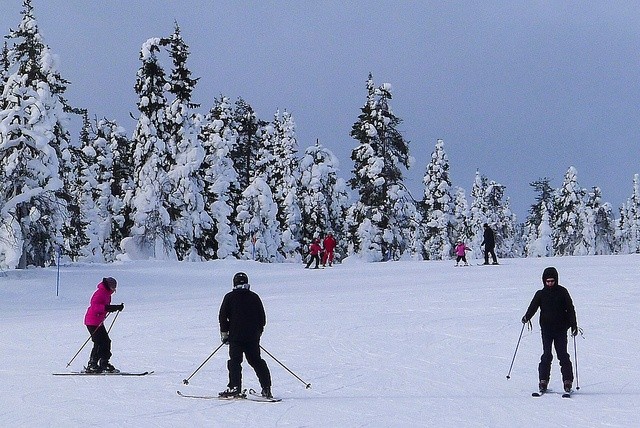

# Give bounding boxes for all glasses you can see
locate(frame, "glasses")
[546,280,554,282]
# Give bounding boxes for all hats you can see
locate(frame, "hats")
[328,230,332,234]
[456,240,462,243]
[234,272,248,287]
[107,277,117,288]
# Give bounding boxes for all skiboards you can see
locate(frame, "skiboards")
[477,264,509,266]
[310,268,324,269]
[177,391,282,402]
[52,371,154,376]
[454,265,473,267]
[532,389,574,398]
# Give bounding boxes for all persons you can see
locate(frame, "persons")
[522,266,579,392]
[219,272,273,398]
[455,240,472,265]
[481,223,498,265]
[83,276,123,374]
[321,232,336,267]
[305,238,324,268]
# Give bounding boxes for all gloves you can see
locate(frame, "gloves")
[221,332,230,344]
[106,305,118,311]
[117,304,124,311]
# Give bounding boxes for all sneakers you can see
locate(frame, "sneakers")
[219,386,239,397]
[101,360,120,373]
[539,380,547,392]
[86,362,110,373]
[261,387,271,398]
[564,379,572,392]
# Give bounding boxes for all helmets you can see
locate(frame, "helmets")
[315,238,320,243]
